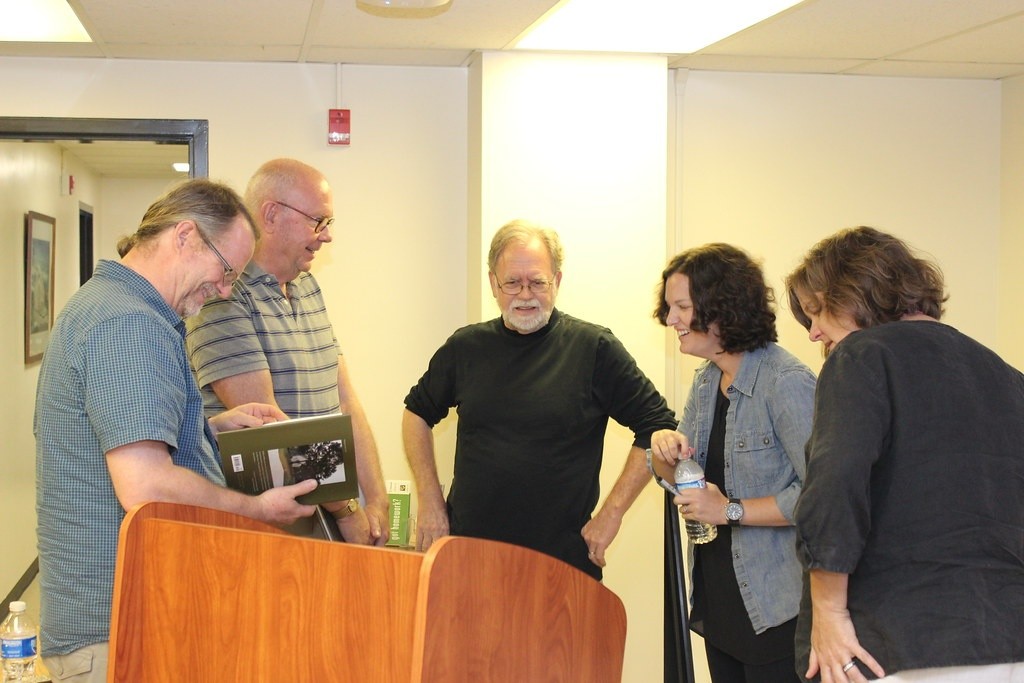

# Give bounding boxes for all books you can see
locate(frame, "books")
[216,413,359,505]
[384,480,411,548]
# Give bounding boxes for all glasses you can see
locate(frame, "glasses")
[175,220,237,287]
[276,201,335,233]
[495,272,555,295]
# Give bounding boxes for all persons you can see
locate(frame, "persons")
[32,182,319,683]
[644,244,818,683]
[185,159,392,549]
[782,226,1024,683]
[401,221,680,582]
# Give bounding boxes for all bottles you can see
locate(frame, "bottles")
[0,601,38,683]
[674,451,717,544]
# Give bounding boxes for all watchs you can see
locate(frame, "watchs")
[330,499,359,521]
[724,498,745,528]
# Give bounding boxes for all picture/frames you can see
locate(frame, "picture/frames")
[24,211,55,365]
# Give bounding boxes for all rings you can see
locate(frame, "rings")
[588,552,596,555]
[842,661,855,673]
